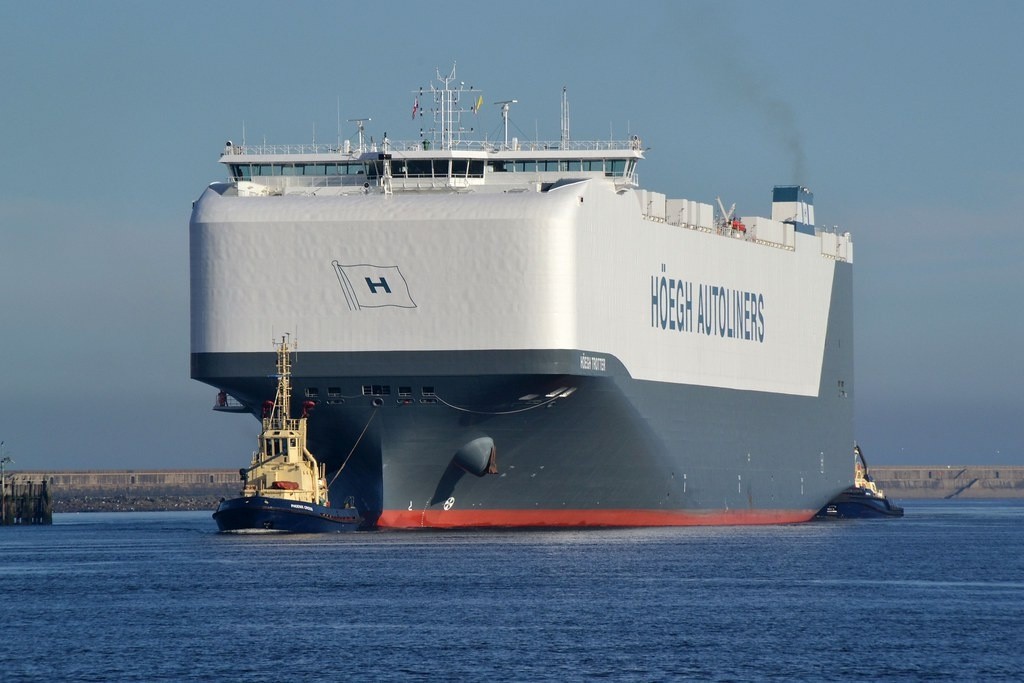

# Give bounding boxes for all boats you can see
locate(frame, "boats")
[817,438,903,521]
[213,320,362,531]
[0,440,55,526]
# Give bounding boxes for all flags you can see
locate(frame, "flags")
[412,97,418,119]
[477,95,483,110]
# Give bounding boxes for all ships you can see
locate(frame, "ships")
[188,59,856,531]
